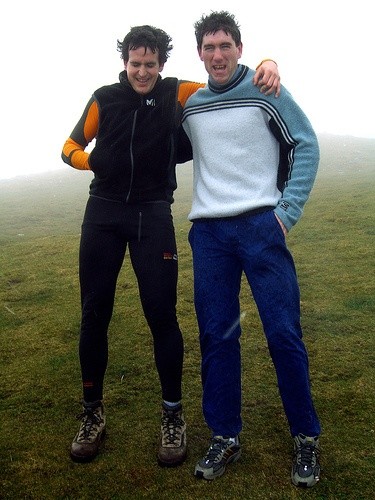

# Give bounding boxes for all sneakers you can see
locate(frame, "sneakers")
[157,401,188,467]
[194,433,243,482]
[289,430,322,490]
[68,401,107,464]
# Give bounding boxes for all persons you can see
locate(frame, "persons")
[175,12,324,489]
[60,26,283,469]
